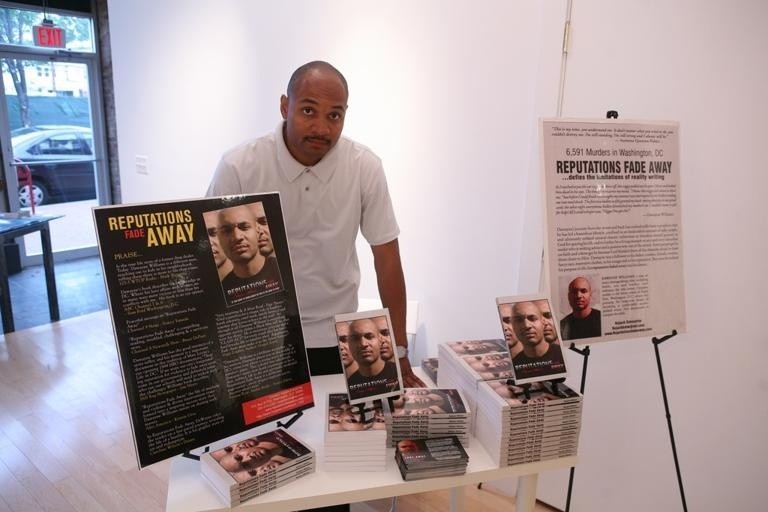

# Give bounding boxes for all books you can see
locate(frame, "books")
[199,426,315,508]
[321,386,472,481]
[422,338,584,469]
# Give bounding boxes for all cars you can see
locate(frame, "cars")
[0,125,96,208]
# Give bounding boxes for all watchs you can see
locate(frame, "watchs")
[395,346,408,360]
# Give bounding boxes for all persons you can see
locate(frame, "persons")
[369,315,396,364]
[334,321,359,380]
[204,61,429,510]
[497,303,524,360]
[561,276,601,340]
[511,301,566,380]
[347,318,400,400]
[529,298,560,346]
[244,201,276,259]
[216,204,286,306]
[202,211,233,283]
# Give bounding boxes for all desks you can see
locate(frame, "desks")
[0,212,67,334]
[166,366,577,512]
